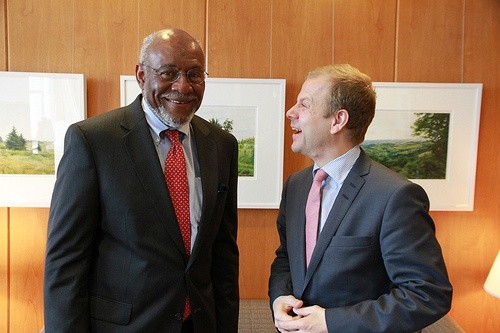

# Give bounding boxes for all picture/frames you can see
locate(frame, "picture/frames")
[0,71,87,209]
[360,82,483,211]
[119,75,286,209]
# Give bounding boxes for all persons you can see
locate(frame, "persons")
[268,63,454,333]
[43,28,239,333]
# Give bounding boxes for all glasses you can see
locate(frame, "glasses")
[143,64,209,85]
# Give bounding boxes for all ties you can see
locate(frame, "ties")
[305,169,329,273]
[161,129,191,321]
